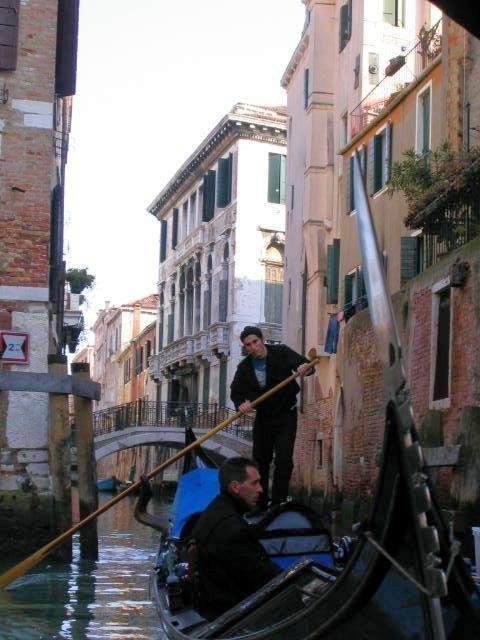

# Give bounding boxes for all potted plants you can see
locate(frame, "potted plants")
[65,268,94,293]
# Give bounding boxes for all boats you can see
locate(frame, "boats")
[132,146,477,640]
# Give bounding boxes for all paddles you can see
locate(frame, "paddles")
[0,356,321,592]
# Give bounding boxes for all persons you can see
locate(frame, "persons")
[230,326,316,518]
[190,457,272,622]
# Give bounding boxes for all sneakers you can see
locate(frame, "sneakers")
[244,501,268,519]
[269,501,281,511]
